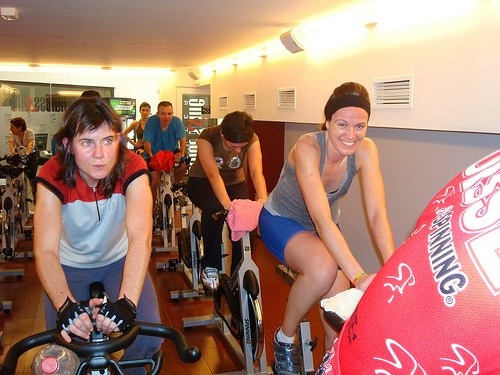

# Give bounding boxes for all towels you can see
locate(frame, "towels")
[149,150,175,173]
[319,287,364,321]
[225,198,264,242]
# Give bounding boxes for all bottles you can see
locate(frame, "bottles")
[31,344,81,375]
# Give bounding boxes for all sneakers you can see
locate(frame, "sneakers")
[202,266,219,291]
[273,326,300,375]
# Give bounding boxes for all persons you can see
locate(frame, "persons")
[142,101,186,224]
[187,111,267,291]
[32,98,164,375]
[121,102,151,157]
[8,117,40,205]
[258,82,395,375]
[51,90,127,156]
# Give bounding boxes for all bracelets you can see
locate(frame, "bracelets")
[353,272,367,285]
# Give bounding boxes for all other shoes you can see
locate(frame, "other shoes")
[175,187,183,197]
